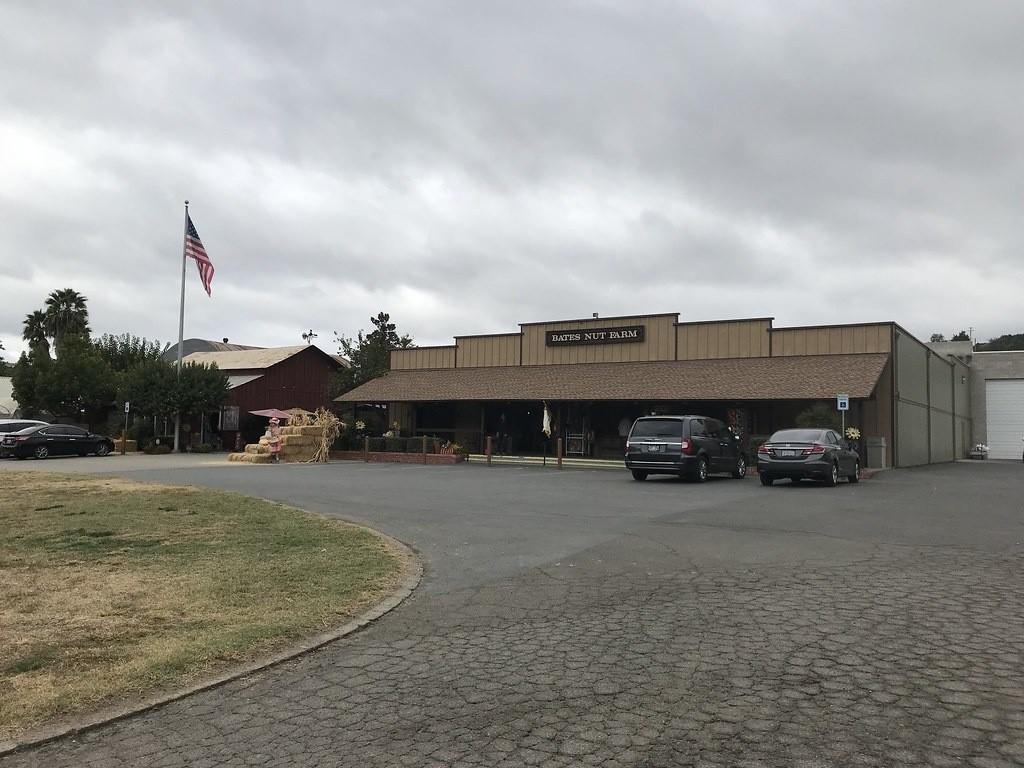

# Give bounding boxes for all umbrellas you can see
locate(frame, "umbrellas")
[250,408,292,420]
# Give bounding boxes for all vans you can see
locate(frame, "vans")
[0,419,51,445]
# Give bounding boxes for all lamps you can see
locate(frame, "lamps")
[592,313,599,318]
[962,376,966,384]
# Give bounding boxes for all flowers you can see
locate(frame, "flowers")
[846,427,860,449]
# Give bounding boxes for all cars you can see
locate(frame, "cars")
[0,423,116,460]
[756,428,861,486]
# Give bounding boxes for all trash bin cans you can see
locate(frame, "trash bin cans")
[865,436,887,468]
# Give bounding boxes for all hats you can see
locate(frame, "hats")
[269,418,280,424]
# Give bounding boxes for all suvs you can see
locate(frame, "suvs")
[625,413,747,483]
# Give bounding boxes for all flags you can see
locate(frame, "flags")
[184,216,215,297]
[541,406,552,440]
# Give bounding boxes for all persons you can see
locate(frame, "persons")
[268,417,281,460]
[618,415,631,458]
